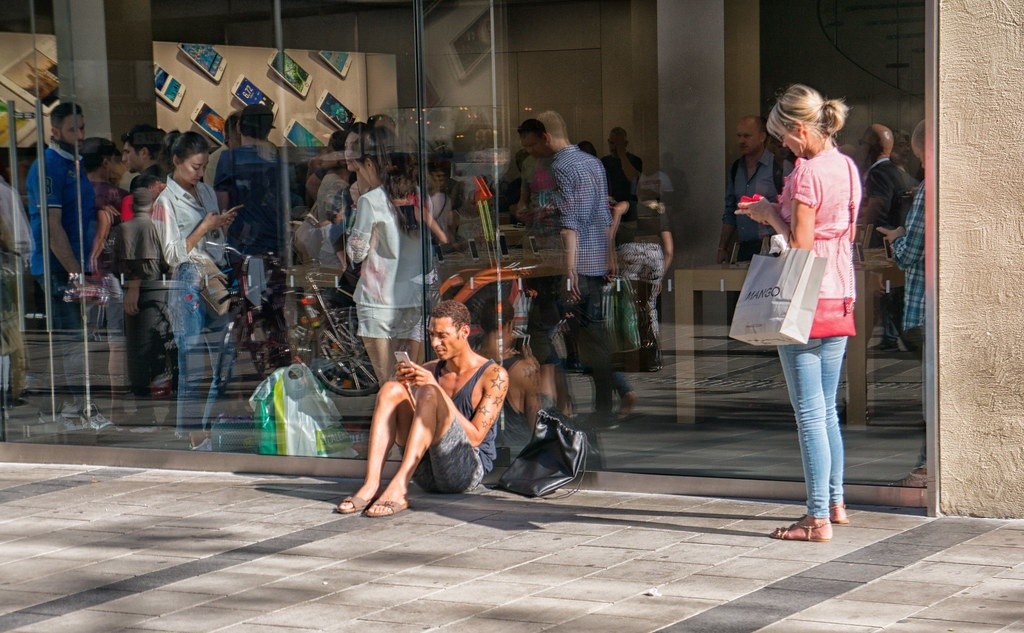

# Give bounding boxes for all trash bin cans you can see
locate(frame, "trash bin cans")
[125,281,192,400]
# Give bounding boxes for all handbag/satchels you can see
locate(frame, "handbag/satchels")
[494,407,588,500]
[194,256,232,318]
[728,235,828,345]
[247,362,360,458]
[63,268,110,305]
[601,274,641,353]
[209,414,259,453]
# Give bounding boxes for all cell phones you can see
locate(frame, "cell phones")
[855,224,893,263]
[317,51,353,79]
[267,49,313,98]
[153,62,186,110]
[435,232,568,264]
[177,43,228,83]
[394,352,412,368]
[316,89,361,131]
[231,74,278,119]
[446,7,492,81]
[283,118,327,146]
[226,204,244,214]
[730,242,740,265]
[190,100,226,146]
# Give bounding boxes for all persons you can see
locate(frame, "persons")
[203,103,282,276]
[333,299,509,518]
[81,123,165,432]
[303,113,496,387]
[0,176,35,408]
[473,298,542,473]
[528,109,638,434]
[503,118,555,223]
[110,188,170,425]
[734,84,862,542]
[609,173,672,371]
[860,123,924,367]
[575,126,643,246]
[876,123,926,488]
[26,103,96,422]
[151,128,240,451]
[717,114,793,377]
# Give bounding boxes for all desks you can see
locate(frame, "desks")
[438,211,572,303]
[673,247,905,427]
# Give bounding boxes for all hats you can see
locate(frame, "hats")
[242,102,276,129]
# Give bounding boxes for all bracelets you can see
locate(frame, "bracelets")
[719,247,727,251]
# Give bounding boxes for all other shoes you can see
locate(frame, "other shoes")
[58,411,79,427]
[868,337,899,351]
[189,436,213,453]
[79,406,115,430]
[614,391,638,422]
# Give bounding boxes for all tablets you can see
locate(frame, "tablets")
[0,37,61,115]
[0,97,37,147]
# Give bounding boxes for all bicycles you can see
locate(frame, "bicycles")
[203,239,379,396]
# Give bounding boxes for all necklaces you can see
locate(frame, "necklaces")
[357,180,371,196]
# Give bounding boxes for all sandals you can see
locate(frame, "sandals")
[889,466,928,487]
[801,504,850,523]
[768,515,833,543]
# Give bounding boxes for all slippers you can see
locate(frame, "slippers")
[335,492,380,513]
[366,497,411,517]
